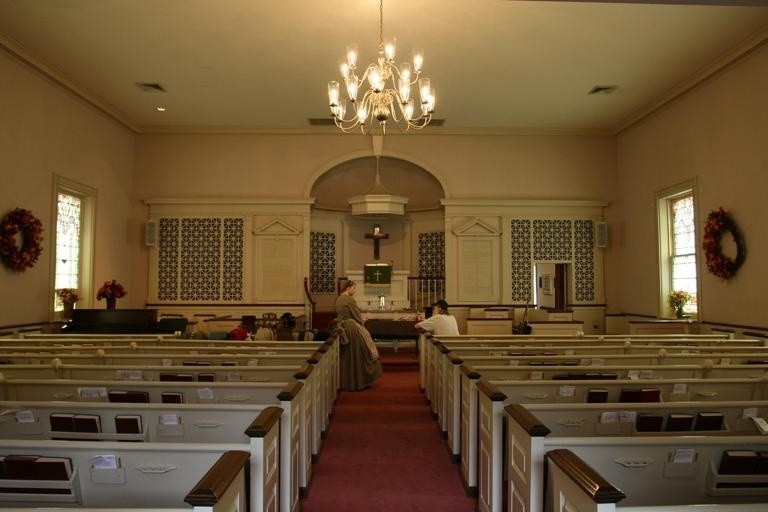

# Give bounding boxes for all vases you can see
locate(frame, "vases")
[63,303,74,320]
[675,304,685,319]
[106,298,116,310]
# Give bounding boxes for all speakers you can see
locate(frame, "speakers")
[144,220,156,247]
[596,221,608,248]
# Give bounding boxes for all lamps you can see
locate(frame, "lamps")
[346,156,409,216]
[328,0,435,136]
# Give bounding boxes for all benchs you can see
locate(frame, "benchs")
[417,328,768,512]
[0,331,340,512]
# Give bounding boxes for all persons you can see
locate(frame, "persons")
[414,299,460,335]
[334,280,384,391]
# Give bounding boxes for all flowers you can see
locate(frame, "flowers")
[0,206,45,273]
[96,278,127,301]
[55,288,83,305]
[667,291,694,312]
[702,206,746,282]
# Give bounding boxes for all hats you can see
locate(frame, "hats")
[430,299,448,309]
